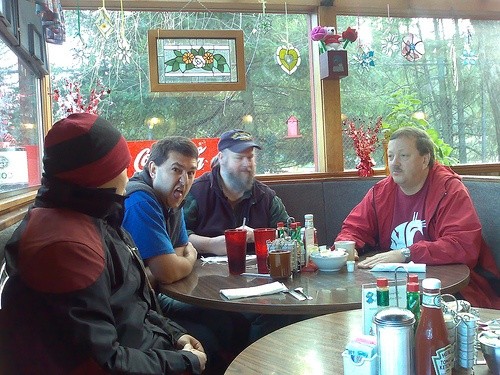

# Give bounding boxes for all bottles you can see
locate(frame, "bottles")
[371,307,416,375]
[376,278,389,311]
[406,274,421,331]
[303,214,318,271]
[276,221,306,273]
[414,278,453,375]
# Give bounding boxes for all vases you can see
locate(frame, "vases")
[319,49,348,80]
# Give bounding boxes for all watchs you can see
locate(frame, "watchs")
[401,248,410,263]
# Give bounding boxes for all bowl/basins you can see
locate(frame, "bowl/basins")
[310,251,349,271]
[477,328,500,375]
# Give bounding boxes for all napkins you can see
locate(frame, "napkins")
[200,253,256,260]
[371,263,427,272]
[219,281,284,300]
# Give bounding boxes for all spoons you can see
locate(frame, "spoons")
[294,287,313,300]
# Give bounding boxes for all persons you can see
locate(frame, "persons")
[122,136,251,375]
[181,129,324,345]
[331,127,500,310]
[1,113,208,375]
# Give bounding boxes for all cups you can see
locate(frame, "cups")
[224,229,247,275]
[342,350,377,375]
[253,228,276,274]
[268,249,292,280]
[334,241,356,265]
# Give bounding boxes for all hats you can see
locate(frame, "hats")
[42,112,131,189]
[218,130,261,153]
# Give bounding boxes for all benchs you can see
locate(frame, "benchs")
[1,175,500,273]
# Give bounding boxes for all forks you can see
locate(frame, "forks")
[280,283,306,301]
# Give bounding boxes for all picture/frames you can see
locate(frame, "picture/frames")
[148,30,246,92]
[28,24,45,65]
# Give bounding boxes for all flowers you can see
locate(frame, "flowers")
[311,25,359,53]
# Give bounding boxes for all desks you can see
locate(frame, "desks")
[225,307,500,375]
[157,251,469,314]
